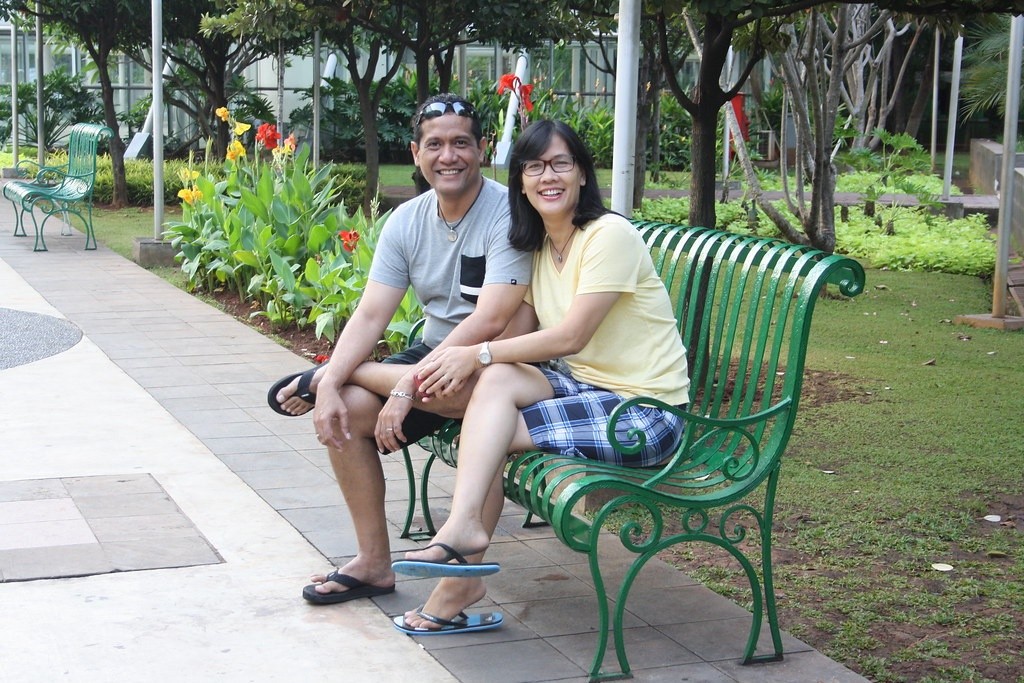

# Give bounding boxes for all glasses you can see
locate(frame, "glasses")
[416,101,474,124]
[523,154,575,177]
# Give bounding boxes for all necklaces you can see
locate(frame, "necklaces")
[439,172,484,242]
[550,227,576,262]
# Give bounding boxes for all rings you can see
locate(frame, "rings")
[316,433,320,437]
[385,428,393,431]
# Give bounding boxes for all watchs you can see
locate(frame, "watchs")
[479,341,492,367]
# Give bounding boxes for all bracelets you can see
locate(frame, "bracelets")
[389,391,416,401]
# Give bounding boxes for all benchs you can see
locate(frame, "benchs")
[403,216,865,683]
[3,121,116,253]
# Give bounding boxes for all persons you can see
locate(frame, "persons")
[267,97,533,603]
[393,117,691,636]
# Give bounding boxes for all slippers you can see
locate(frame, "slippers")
[268,359,329,416]
[302,570,395,604]
[391,543,500,578]
[393,604,504,635]
